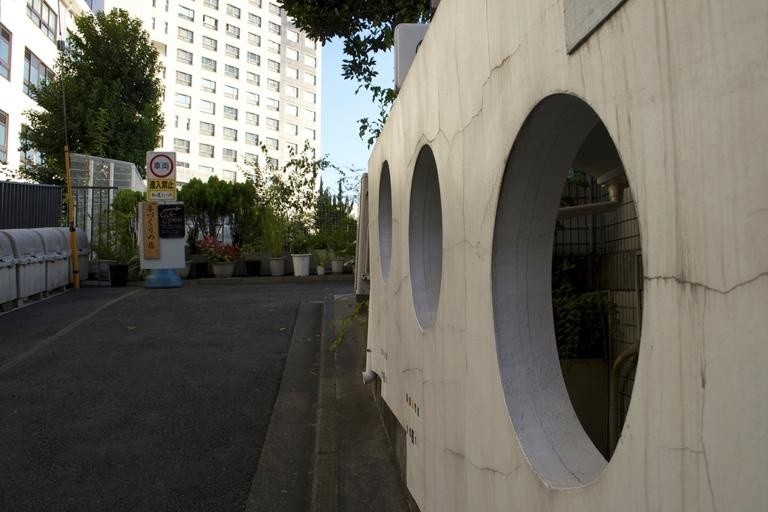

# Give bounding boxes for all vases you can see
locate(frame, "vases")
[212,261,234,277]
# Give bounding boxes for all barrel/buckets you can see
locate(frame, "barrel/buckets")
[245,260,261,275]
[291,254,312,276]
[332,260,344,273]
[270,257,285,276]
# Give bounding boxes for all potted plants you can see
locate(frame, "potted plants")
[90,234,116,281]
[267,223,346,277]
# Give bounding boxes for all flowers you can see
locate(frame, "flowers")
[200,236,240,264]
